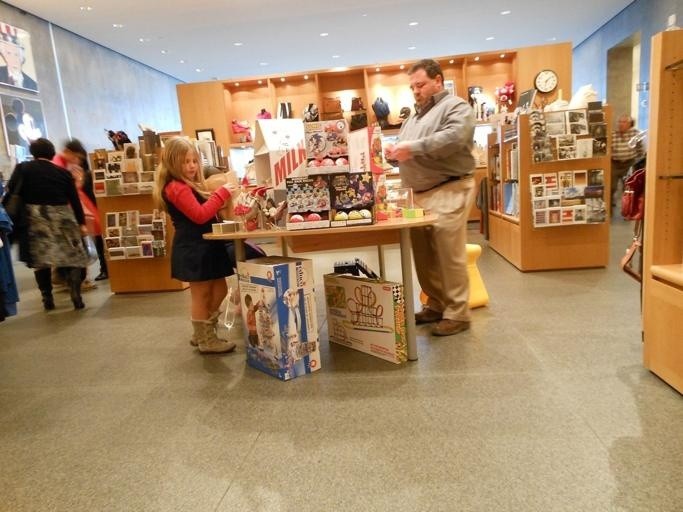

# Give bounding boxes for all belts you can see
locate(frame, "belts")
[408,171,472,195]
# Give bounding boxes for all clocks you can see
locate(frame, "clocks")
[532,68,559,95]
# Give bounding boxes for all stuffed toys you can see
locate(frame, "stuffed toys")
[496,81,515,107]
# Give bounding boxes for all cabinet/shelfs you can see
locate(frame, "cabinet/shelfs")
[85,144,188,294]
[485,110,612,273]
[175,42,571,222]
[641,27,683,401]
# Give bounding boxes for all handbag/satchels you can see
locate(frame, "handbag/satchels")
[5,162,26,216]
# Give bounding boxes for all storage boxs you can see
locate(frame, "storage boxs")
[235,254,321,381]
[323,273,409,364]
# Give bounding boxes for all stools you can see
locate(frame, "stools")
[421,245,491,311]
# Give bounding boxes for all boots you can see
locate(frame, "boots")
[191,320,197,346]
[34,268,56,312]
[68,266,86,310]
[191,319,236,354]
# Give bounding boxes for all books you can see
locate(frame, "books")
[102,235,120,249]
[568,107,589,135]
[492,147,501,180]
[104,212,119,227]
[535,208,547,227]
[124,246,143,258]
[105,226,121,236]
[503,124,518,141]
[151,228,164,241]
[543,173,557,186]
[137,213,155,225]
[104,178,121,196]
[135,235,155,246]
[576,137,593,158]
[141,170,154,193]
[588,168,605,183]
[592,123,608,156]
[560,185,586,206]
[573,204,587,224]
[490,182,502,212]
[121,170,140,194]
[560,207,574,226]
[505,142,519,180]
[573,169,588,186]
[91,148,108,170]
[151,220,166,230]
[532,185,547,198]
[546,194,561,207]
[140,240,154,257]
[587,100,603,118]
[544,111,567,137]
[555,133,576,160]
[122,225,138,236]
[557,170,573,188]
[106,247,126,260]
[588,110,603,133]
[547,188,560,196]
[123,142,139,160]
[136,225,154,236]
[91,169,106,181]
[151,239,166,257]
[105,161,121,180]
[585,184,606,223]
[530,173,541,185]
[504,180,521,219]
[532,198,548,214]
[93,180,106,197]
[126,211,141,227]
[116,211,130,228]
[548,207,562,227]
[118,236,137,246]
[107,151,124,162]
[121,158,143,175]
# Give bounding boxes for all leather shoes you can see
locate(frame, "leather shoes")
[95,270,108,280]
[432,317,472,338]
[414,308,442,325]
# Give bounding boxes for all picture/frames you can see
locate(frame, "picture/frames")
[0,93,53,159]
[0,20,41,95]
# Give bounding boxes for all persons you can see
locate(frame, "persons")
[385,58,476,337]
[610,114,648,209]
[0,22,37,91]
[243,293,261,349]
[59,137,110,281]
[5,137,87,313]
[153,134,237,356]
